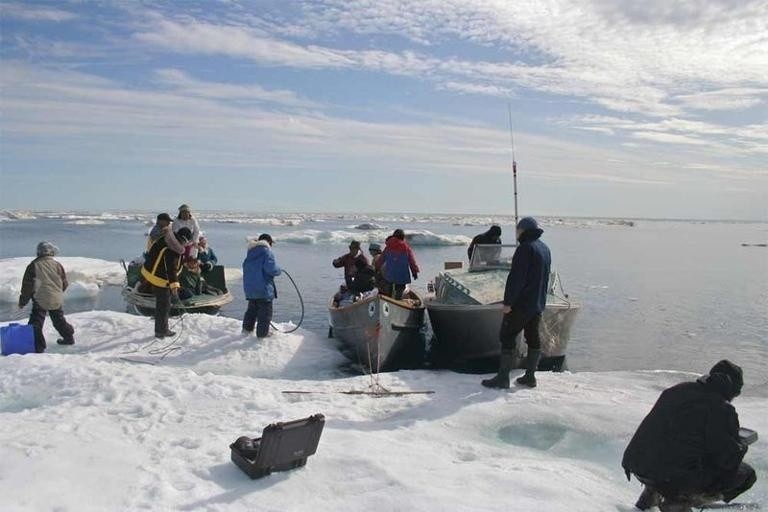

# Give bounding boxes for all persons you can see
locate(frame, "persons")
[621,358,757,512]
[331,228,420,308]
[480,218,552,389]
[241,233,282,338]
[140,226,193,339]
[468,224,503,264]
[17,240,75,354]
[138,205,218,300]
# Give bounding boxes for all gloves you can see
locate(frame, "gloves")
[200,264,211,272]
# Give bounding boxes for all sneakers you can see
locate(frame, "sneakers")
[57,336,75,345]
[242,328,276,340]
[155,330,176,338]
[658,497,694,512]
[635,484,663,510]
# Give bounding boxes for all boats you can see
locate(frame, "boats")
[423,104,574,373]
[117,256,233,317]
[327,278,428,373]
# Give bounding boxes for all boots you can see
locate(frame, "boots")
[482,349,517,389]
[517,347,543,387]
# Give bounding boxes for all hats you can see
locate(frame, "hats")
[37,242,58,255]
[157,213,174,222]
[710,360,744,392]
[199,236,207,241]
[369,243,382,251]
[178,204,190,212]
[351,240,361,248]
[177,227,193,243]
[517,216,539,230]
[258,233,276,245]
